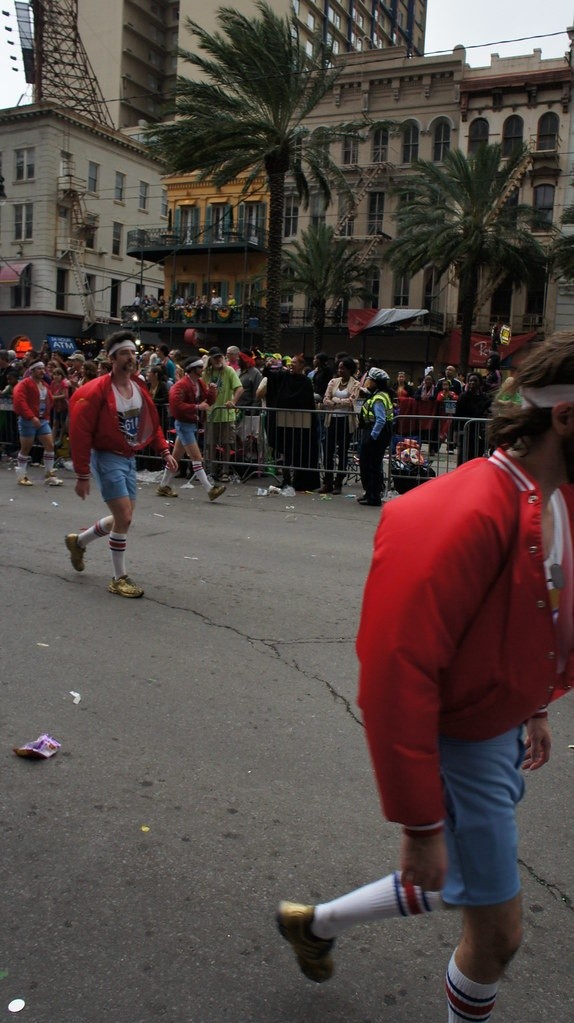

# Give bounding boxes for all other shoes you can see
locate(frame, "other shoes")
[332,488,342,494]
[359,497,382,506]
[318,485,332,493]
[221,472,229,482]
[174,472,187,478]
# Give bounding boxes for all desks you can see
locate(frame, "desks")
[160,234,180,246]
[223,230,242,242]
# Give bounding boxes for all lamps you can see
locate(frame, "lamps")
[15,251,23,259]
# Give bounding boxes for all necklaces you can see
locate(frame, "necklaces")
[340,380,348,385]
[540,502,564,589]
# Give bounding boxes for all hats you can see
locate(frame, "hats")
[68,354,85,362]
[368,366,391,381]
[209,348,223,357]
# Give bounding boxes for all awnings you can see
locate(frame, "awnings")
[0,263,31,287]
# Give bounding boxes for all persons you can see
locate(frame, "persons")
[13,358,64,486]
[135,343,500,494]
[0,340,110,461]
[158,356,227,502]
[64,330,179,598]
[355,366,394,507]
[132,292,237,324]
[277,326,574,1023]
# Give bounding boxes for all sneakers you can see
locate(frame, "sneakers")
[208,486,226,500]
[157,486,178,498]
[277,901,333,984]
[16,477,33,486]
[65,533,85,572]
[44,476,64,486]
[108,575,144,598]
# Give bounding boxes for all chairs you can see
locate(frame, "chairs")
[145,233,159,246]
[383,435,436,494]
[216,416,267,480]
[333,442,361,486]
